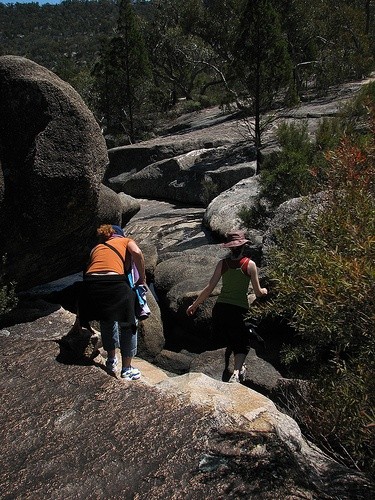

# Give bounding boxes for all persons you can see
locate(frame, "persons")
[187,232,267,385]
[83,227,146,380]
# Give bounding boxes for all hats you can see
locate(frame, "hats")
[111,225,124,235]
[223,230,249,248]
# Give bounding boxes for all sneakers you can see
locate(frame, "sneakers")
[229,363,248,383]
[106,355,118,368]
[120,367,141,380]
